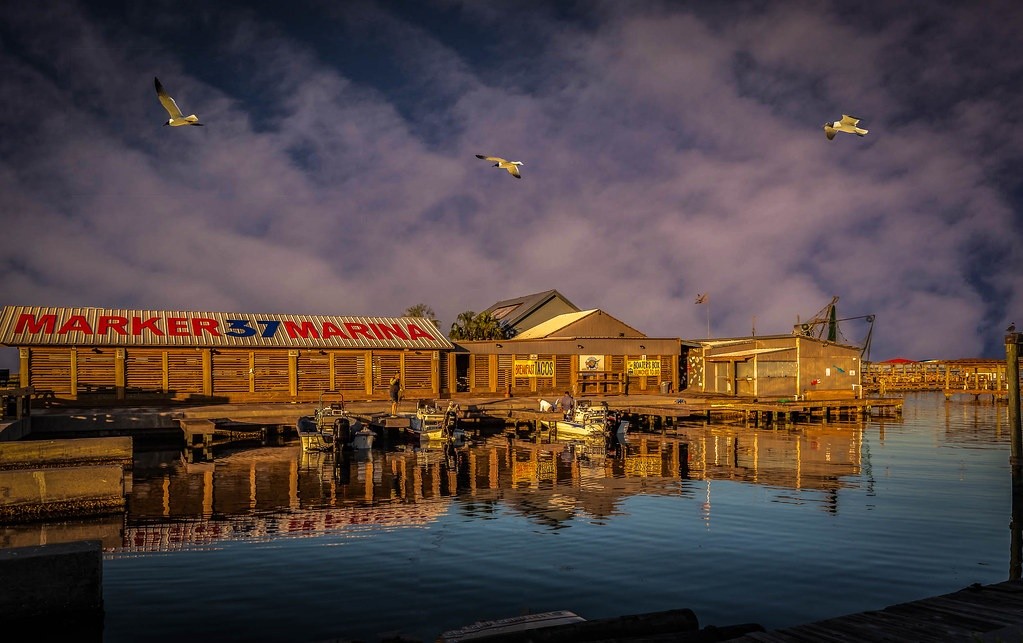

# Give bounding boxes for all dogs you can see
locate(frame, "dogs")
[537,399,554,414]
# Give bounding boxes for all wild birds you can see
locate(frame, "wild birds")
[475,154,524,179]
[154,76,205,128]
[823,115,868,140]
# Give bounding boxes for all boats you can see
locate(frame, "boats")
[295,392,378,459]
[543,397,640,448]
[411,397,498,449]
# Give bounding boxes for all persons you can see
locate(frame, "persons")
[537,399,553,413]
[390,373,401,415]
[561,391,573,420]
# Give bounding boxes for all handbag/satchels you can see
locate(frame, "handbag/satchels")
[567,410,572,415]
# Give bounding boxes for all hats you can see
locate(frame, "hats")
[395,371,400,374]
[537,399,541,402]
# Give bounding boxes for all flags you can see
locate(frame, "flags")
[696,292,709,305]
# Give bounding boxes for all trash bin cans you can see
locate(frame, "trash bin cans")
[793,395,797,401]
[660,381,668,393]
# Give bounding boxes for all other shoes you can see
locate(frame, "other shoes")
[391,414,397,416]
